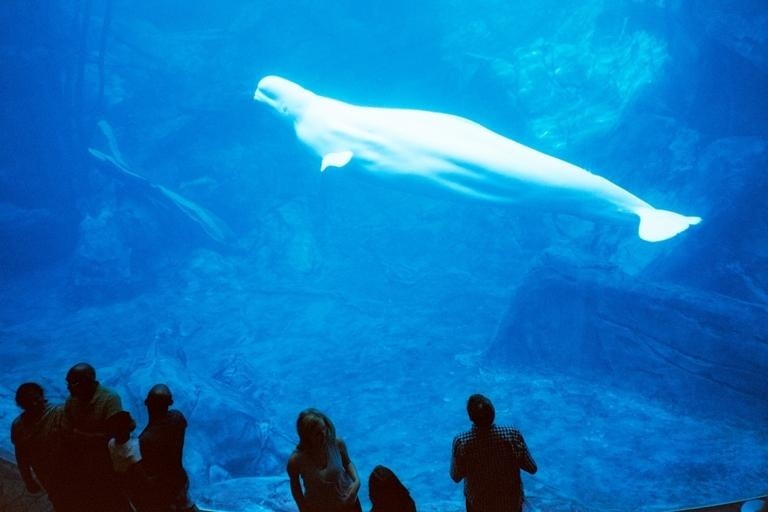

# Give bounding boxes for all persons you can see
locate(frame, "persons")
[11,363,189,512]
[287,408,363,512]
[367,464,416,512]
[449,394,538,511]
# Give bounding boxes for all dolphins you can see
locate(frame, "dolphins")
[252,74,702,244]
